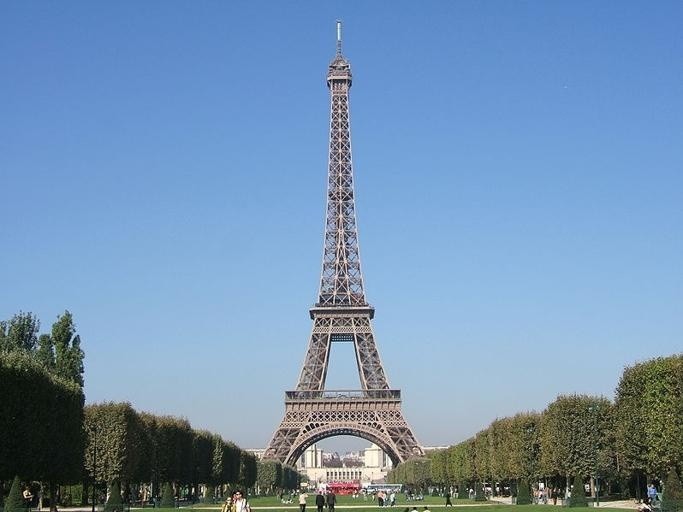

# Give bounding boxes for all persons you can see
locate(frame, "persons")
[568,489,571,498]
[585,486,590,496]
[221,491,250,512]
[107,486,151,503]
[352,488,396,507]
[299,491,308,512]
[638,478,664,512]
[316,487,337,512]
[446,490,452,508]
[469,487,473,498]
[404,485,424,502]
[23,486,34,512]
[277,488,296,504]
[404,506,431,512]
[533,487,558,505]
